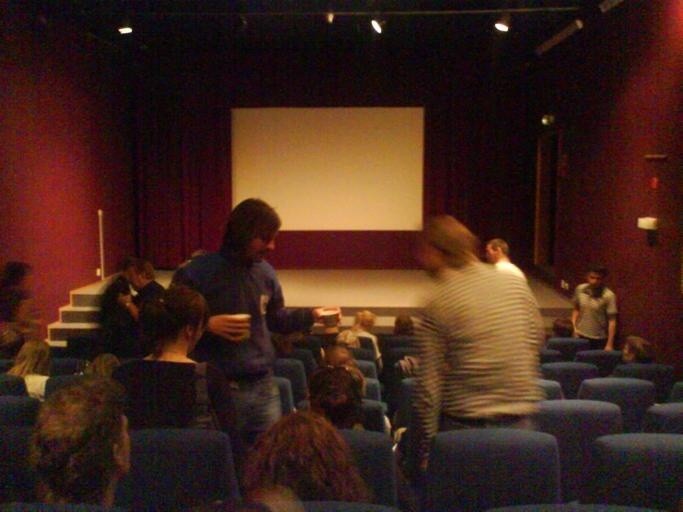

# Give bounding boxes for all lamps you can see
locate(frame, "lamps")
[111,7,579,41]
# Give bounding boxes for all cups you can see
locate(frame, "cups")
[228,314,252,341]
[320,309,338,328]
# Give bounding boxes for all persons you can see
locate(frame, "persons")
[552,318,573,337]
[394,215,544,476]
[220,307,417,511]
[622,336,656,364]
[1,199,285,511]
[570,262,618,351]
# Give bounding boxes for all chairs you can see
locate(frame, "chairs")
[0,329,681,512]
